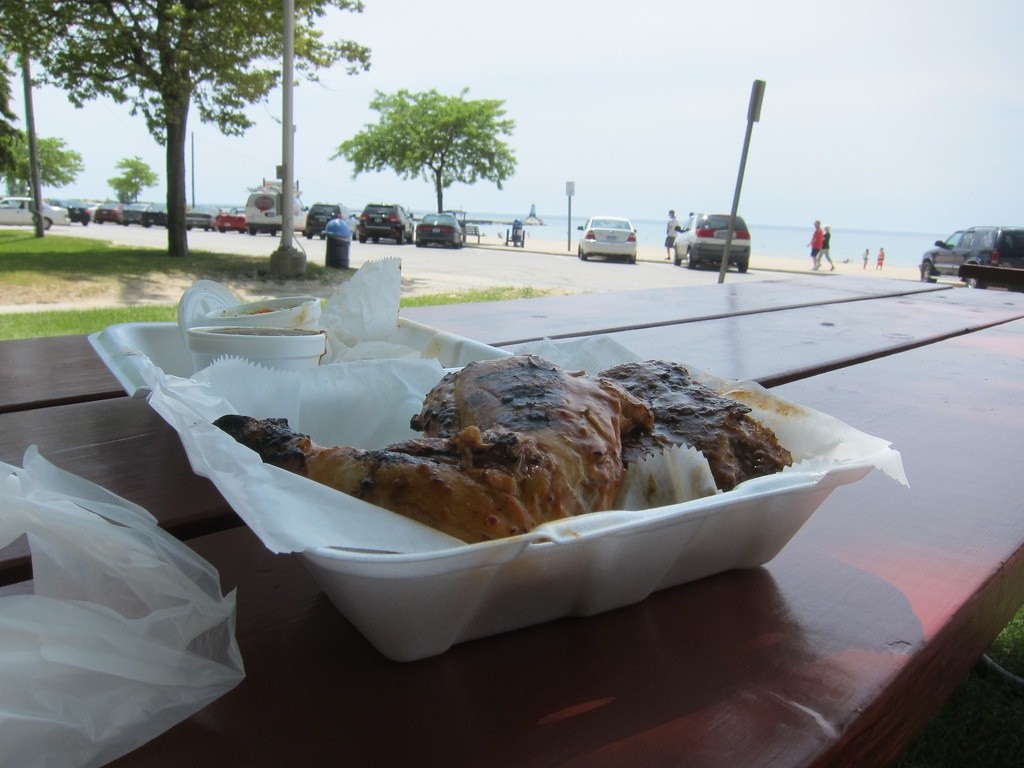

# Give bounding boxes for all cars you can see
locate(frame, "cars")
[0,197,73,231]
[186,205,224,231]
[143,203,170,229]
[87,201,104,222]
[123,203,150,228]
[576,216,638,264]
[49,199,90,226]
[96,203,126,225]
[415,214,463,250]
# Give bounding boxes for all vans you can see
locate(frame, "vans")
[244,188,311,237]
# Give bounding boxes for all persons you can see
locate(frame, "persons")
[876,248,885,270]
[817,226,836,271]
[863,249,869,270]
[807,220,825,270]
[664,210,680,259]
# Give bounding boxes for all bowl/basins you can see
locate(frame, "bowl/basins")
[198,295,325,338]
[186,322,330,381]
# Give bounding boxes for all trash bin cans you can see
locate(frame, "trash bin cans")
[320,218,352,268]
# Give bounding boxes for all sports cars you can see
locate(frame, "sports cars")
[216,207,250,233]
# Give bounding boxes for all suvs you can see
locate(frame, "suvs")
[919,225,1024,295]
[671,213,752,272]
[357,202,416,245]
[305,202,360,241]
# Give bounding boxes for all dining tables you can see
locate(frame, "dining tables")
[1,275,1024,768]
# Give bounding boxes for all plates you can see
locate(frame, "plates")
[82,315,884,665]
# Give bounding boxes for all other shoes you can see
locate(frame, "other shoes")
[830,266,835,272]
[665,258,671,260]
